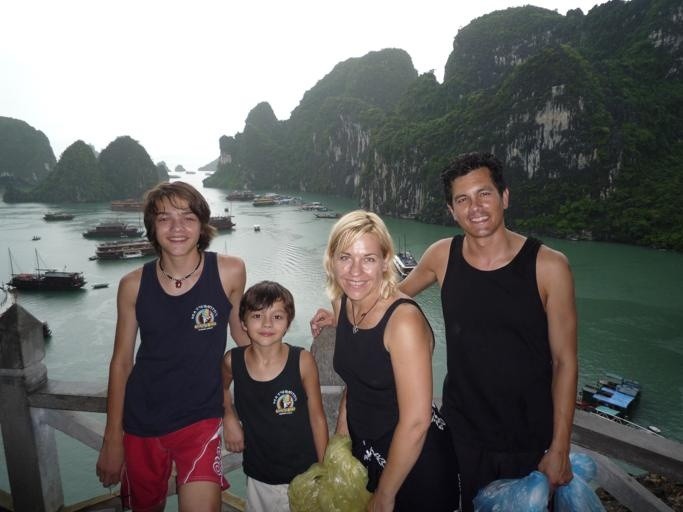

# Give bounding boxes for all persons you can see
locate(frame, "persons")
[309,151,579,512]
[97,181,250,512]
[324,210,460,512]
[222,280,329,512]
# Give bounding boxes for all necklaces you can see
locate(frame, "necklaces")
[160,251,202,288]
[352,297,380,337]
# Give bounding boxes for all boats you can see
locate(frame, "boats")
[43,199,236,260]
[393,232,418,279]
[225,189,341,219]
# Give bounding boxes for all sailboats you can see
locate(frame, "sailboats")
[5,247,88,290]
